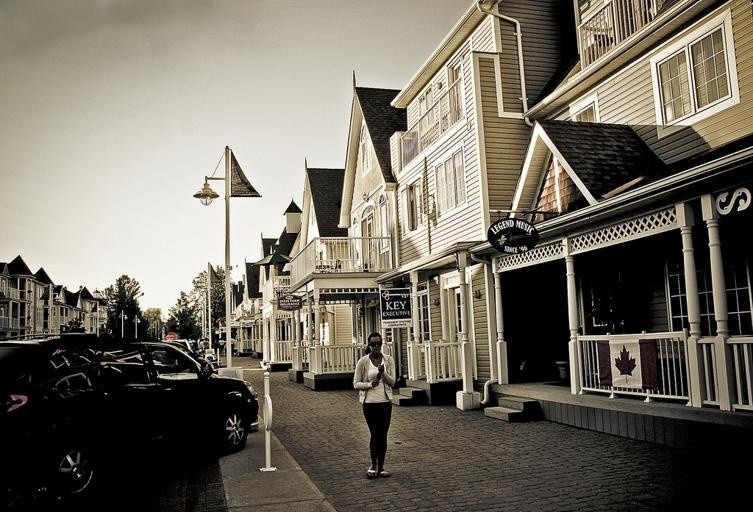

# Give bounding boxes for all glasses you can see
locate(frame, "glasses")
[369,342,382,347]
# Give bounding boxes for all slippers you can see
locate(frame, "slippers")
[368,465,392,478]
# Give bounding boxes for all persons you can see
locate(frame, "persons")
[354,332,396,478]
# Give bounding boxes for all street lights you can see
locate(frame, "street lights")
[194,146,231,368]
